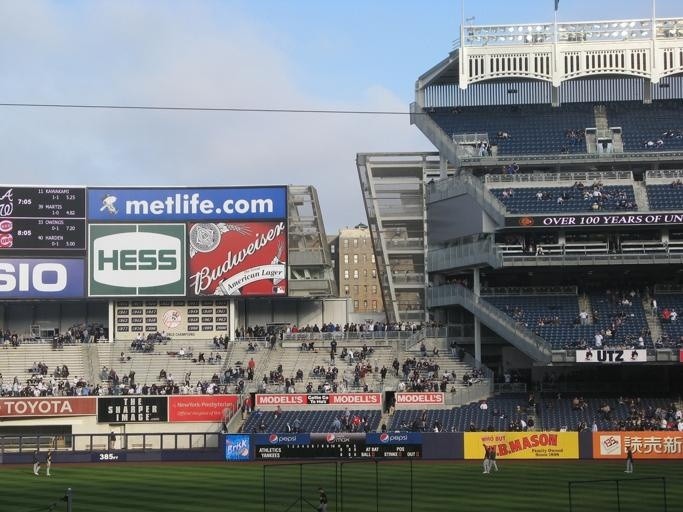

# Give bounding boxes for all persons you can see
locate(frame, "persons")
[108,429,117,451]
[501,178,638,211]
[500,162,520,174]
[481,443,489,475]
[562,128,585,146]
[638,126,682,150]
[495,128,510,140]
[622,446,634,474]
[30,450,41,476]
[476,140,485,156]
[676,179,681,185]
[670,180,676,188]
[486,143,492,156]
[316,486,327,511]
[488,448,498,473]
[43,451,53,477]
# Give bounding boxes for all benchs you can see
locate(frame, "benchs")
[0,338,416,437]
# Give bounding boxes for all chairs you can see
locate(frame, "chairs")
[418,100,682,431]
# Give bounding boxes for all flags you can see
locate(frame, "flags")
[553,1,559,13]
